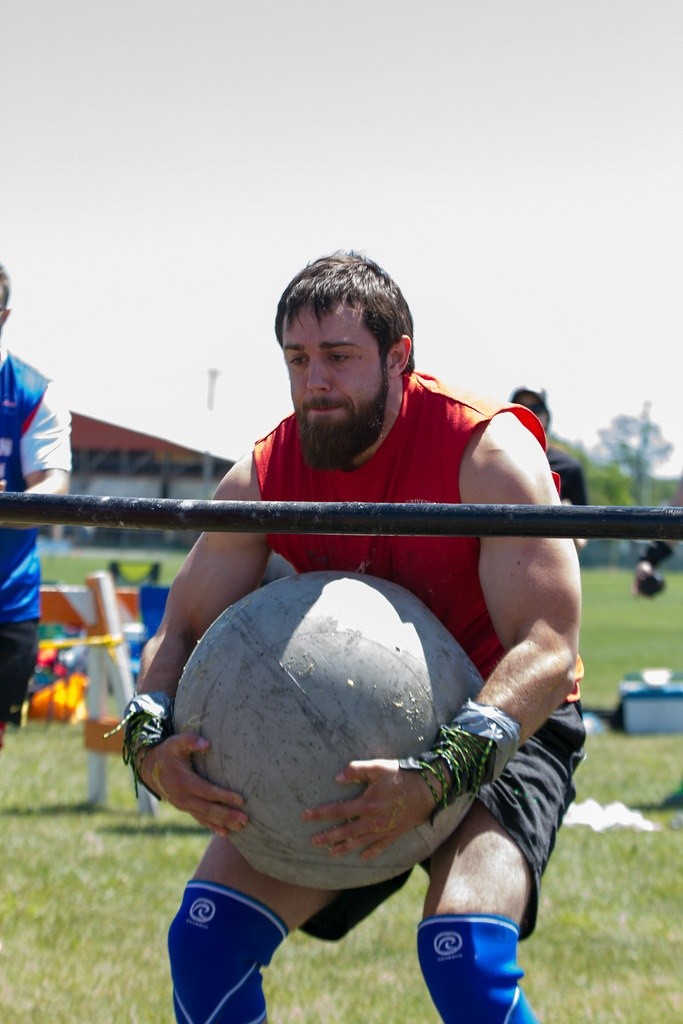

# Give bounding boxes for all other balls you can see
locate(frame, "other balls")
[173,568,492,890]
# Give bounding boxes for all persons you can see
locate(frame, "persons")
[510,385,588,557]
[1,262,73,756]
[124,252,588,1024]
[633,480,683,596]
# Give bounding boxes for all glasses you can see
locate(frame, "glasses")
[520,403,544,416]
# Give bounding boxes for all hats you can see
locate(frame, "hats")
[512,385,550,429]
